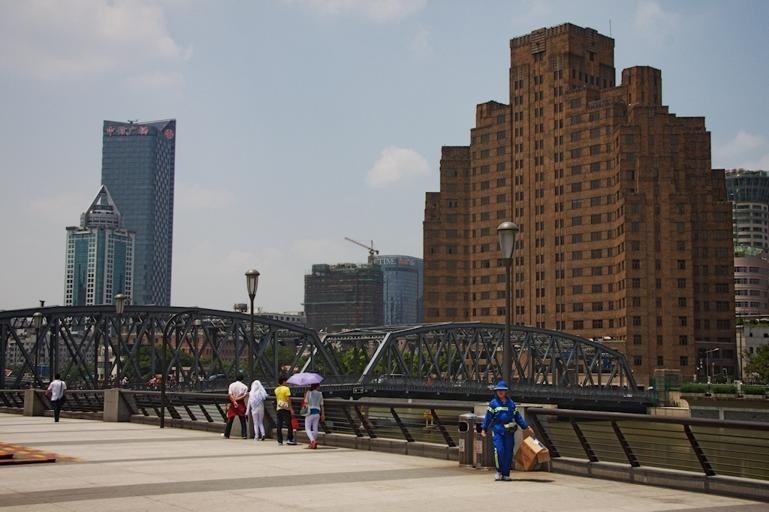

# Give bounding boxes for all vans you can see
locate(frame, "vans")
[453,379,478,389]
[372,373,404,385]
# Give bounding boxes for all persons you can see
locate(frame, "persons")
[274,376,296,445]
[221,373,268,441]
[302,384,325,449]
[481,381,534,480]
[45,373,67,422]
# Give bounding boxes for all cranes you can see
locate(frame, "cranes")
[343,235,380,256]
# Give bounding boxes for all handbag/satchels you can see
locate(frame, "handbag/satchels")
[278,401,289,409]
[58,395,66,405]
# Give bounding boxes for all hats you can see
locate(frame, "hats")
[493,381,509,391]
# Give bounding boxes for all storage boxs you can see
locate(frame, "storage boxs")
[514,434,551,471]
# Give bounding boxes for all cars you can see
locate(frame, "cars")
[203,374,227,389]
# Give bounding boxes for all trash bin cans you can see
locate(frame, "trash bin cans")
[457,413,496,472]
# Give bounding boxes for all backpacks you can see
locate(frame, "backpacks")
[299,407,310,417]
[498,422,518,434]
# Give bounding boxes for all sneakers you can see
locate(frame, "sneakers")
[220,433,266,442]
[493,473,512,481]
[276,439,319,448]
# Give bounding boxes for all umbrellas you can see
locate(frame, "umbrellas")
[287,372,324,392]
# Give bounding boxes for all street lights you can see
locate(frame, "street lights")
[32,312,43,389]
[589,335,612,387]
[244,267,259,385]
[494,222,518,401]
[706,347,720,382]
[114,293,125,385]
